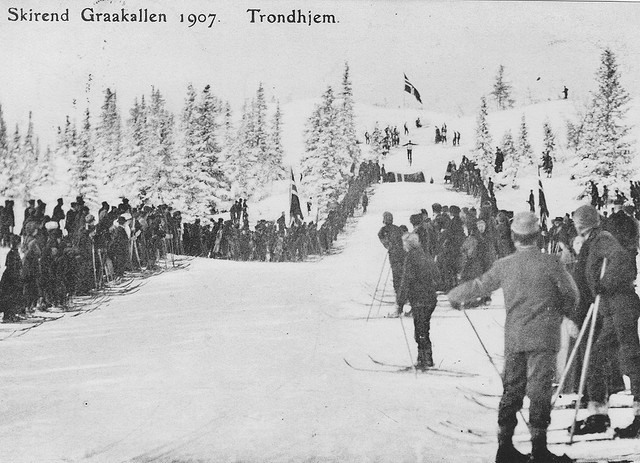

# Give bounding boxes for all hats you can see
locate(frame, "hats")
[510,211,539,234]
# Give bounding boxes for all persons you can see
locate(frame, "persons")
[0,195,182,324]
[377,213,404,306]
[443,156,496,209]
[430,202,517,293]
[540,214,578,254]
[364,132,370,145]
[378,126,400,152]
[287,221,332,262]
[328,160,426,228]
[402,140,418,167]
[453,131,456,145]
[181,198,287,264]
[448,211,576,463]
[398,212,439,258]
[571,205,640,438]
[415,117,421,127]
[457,131,461,144]
[434,123,447,143]
[528,189,535,214]
[590,175,640,253]
[404,121,409,135]
[386,232,438,372]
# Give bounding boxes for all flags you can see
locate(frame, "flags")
[290,170,305,221]
[404,74,424,105]
[538,179,551,218]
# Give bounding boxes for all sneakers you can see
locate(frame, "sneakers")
[614,414,640,437]
[567,414,610,434]
[407,363,426,372]
[531,439,572,463]
[495,443,534,462]
